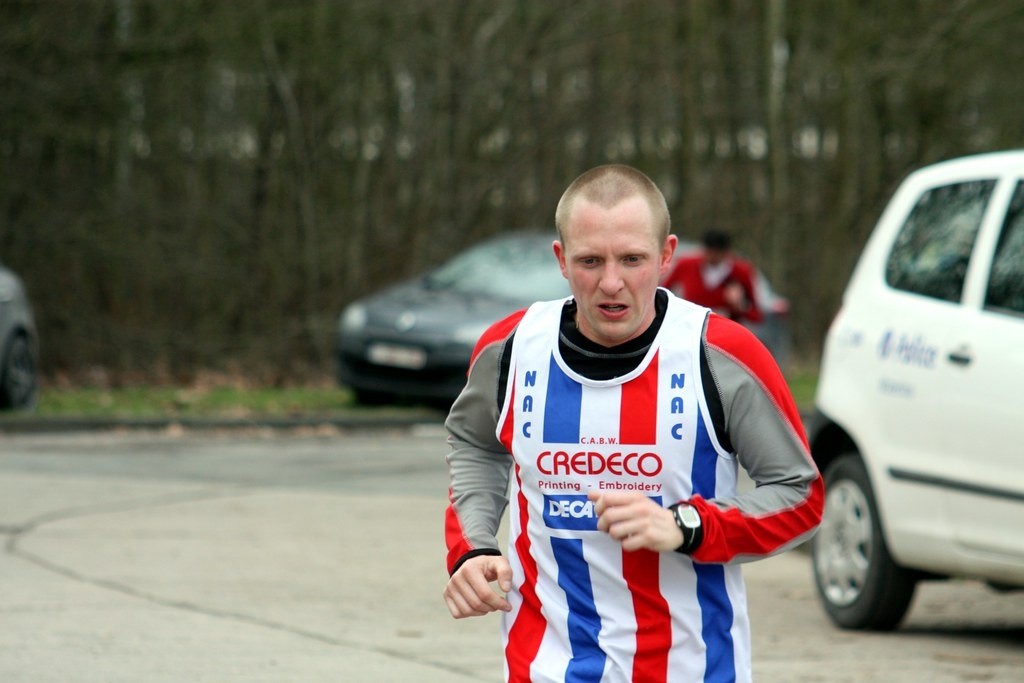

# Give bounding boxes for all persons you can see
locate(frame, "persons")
[443,164,826,683]
[661,228,765,326]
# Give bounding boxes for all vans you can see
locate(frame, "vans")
[804,154,1024,632]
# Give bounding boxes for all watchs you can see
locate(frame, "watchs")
[668,502,704,555]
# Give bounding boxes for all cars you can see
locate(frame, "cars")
[0,267,42,408]
[328,228,703,406]
